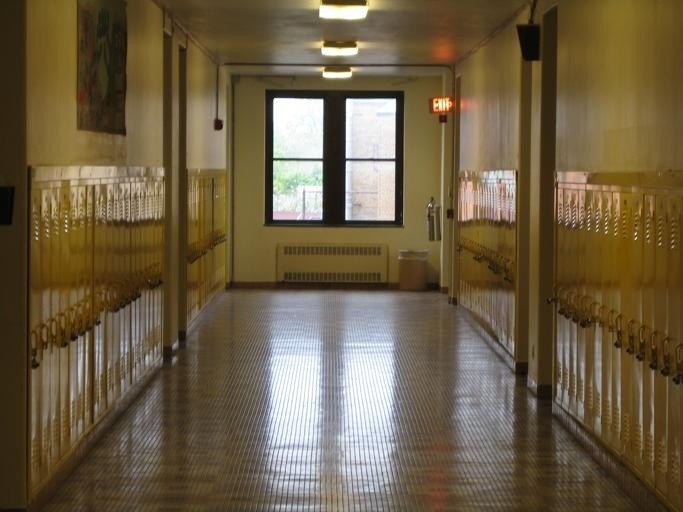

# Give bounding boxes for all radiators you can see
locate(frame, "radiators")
[275,244,388,285]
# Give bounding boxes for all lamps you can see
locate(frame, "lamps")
[321,42,359,59]
[319,1,367,21]
[324,66,352,83]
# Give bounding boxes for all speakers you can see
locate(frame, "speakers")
[515,24,541,60]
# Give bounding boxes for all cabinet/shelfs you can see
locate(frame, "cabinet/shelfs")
[184,170,228,329]
[549,169,680,506]
[453,166,517,358]
[26,164,166,506]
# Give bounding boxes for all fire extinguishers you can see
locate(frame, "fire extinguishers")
[427,196,437,240]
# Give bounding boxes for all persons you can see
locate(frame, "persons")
[90,9,112,97]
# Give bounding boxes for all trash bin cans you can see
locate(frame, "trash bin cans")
[397,249,429,292]
